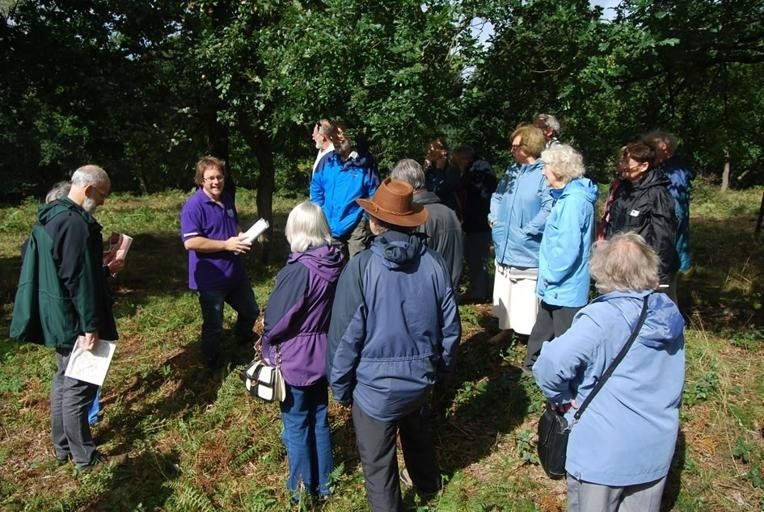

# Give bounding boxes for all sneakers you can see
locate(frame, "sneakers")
[80,449,129,474]
[400,466,443,496]
[509,369,533,379]
[241,335,260,344]
[207,356,224,369]
[488,332,507,343]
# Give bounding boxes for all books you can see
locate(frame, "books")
[232,217,269,255]
[63,335,117,387]
[102,230,133,279]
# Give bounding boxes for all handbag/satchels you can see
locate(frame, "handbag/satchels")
[245,336,286,402]
[537,295,648,480]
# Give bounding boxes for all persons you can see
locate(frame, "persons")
[533,114,561,149]
[598,146,627,240]
[604,142,682,306]
[348,158,462,301]
[637,127,695,286]
[10,164,120,469]
[311,119,382,260]
[507,145,600,381]
[488,126,556,346]
[180,155,260,369]
[532,230,687,512]
[421,134,457,206]
[253,200,345,507]
[325,178,463,512]
[311,119,336,174]
[453,142,498,305]
[44,180,126,428]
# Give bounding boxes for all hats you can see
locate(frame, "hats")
[356,176,428,227]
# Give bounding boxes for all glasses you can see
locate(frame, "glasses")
[96,189,106,199]
[511,144,521,152]
[203,175,224,181]
